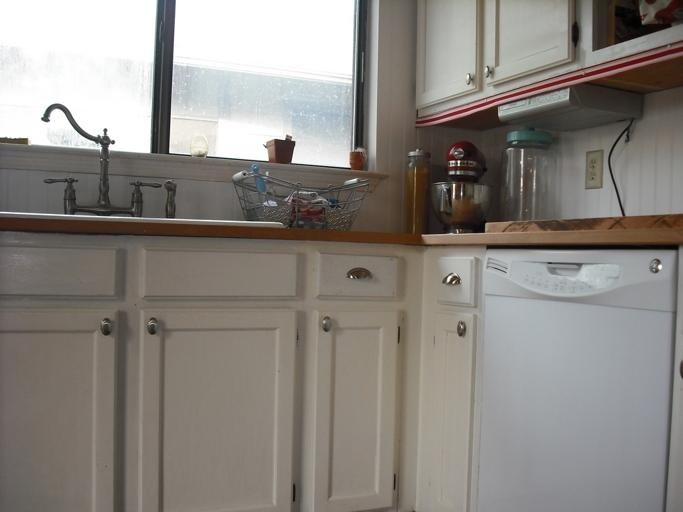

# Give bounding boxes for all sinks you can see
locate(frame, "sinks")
[0,211,283,230]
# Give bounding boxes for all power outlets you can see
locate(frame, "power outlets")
[585,150,603,189]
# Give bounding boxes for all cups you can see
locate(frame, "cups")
[348,151,363,169]
[189,137,208,159]
[265,140,295,163]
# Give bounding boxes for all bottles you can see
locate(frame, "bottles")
[500,129,559,218]
[404,148,430,234]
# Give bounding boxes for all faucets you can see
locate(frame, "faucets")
[40,103,100,145]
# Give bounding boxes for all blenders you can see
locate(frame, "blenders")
[431,141,494,234]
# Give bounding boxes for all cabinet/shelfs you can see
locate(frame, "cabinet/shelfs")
[577,0,683,73]
[413,1,577,119]
[415,245,478,512]
[296,239,407,512]
[0,229,296,508]
